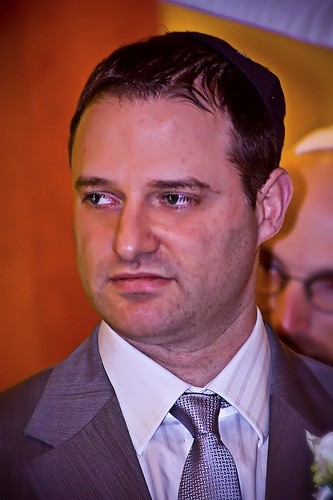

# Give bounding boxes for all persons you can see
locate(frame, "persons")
[0,30,333,500]
[256,122,333,369]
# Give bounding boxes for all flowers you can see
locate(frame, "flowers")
[303,429,333,500]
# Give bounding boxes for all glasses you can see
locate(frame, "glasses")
[252,243,333,315]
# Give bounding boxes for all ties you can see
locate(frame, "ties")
[168,392,243,500]
